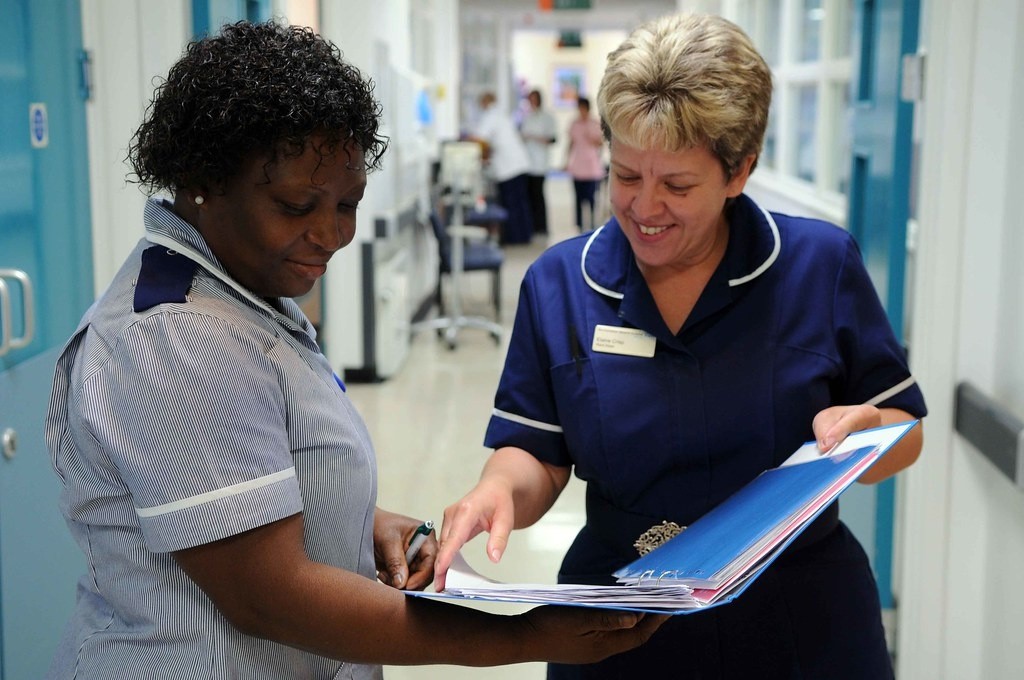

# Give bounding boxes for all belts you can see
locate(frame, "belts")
[585,485,838,567]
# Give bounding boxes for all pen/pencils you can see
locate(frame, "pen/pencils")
[405,519,435,565]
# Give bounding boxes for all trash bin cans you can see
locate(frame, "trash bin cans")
[325,202,442,385]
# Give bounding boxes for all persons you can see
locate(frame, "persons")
[42,18,668,680]
[435,13,928,680]
[518,91,558,235]
[564,97,606,234]
[469,89,537,245]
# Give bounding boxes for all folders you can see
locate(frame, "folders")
[397,417,920,616]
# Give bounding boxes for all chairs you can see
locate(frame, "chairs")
[418,185,507,342]
